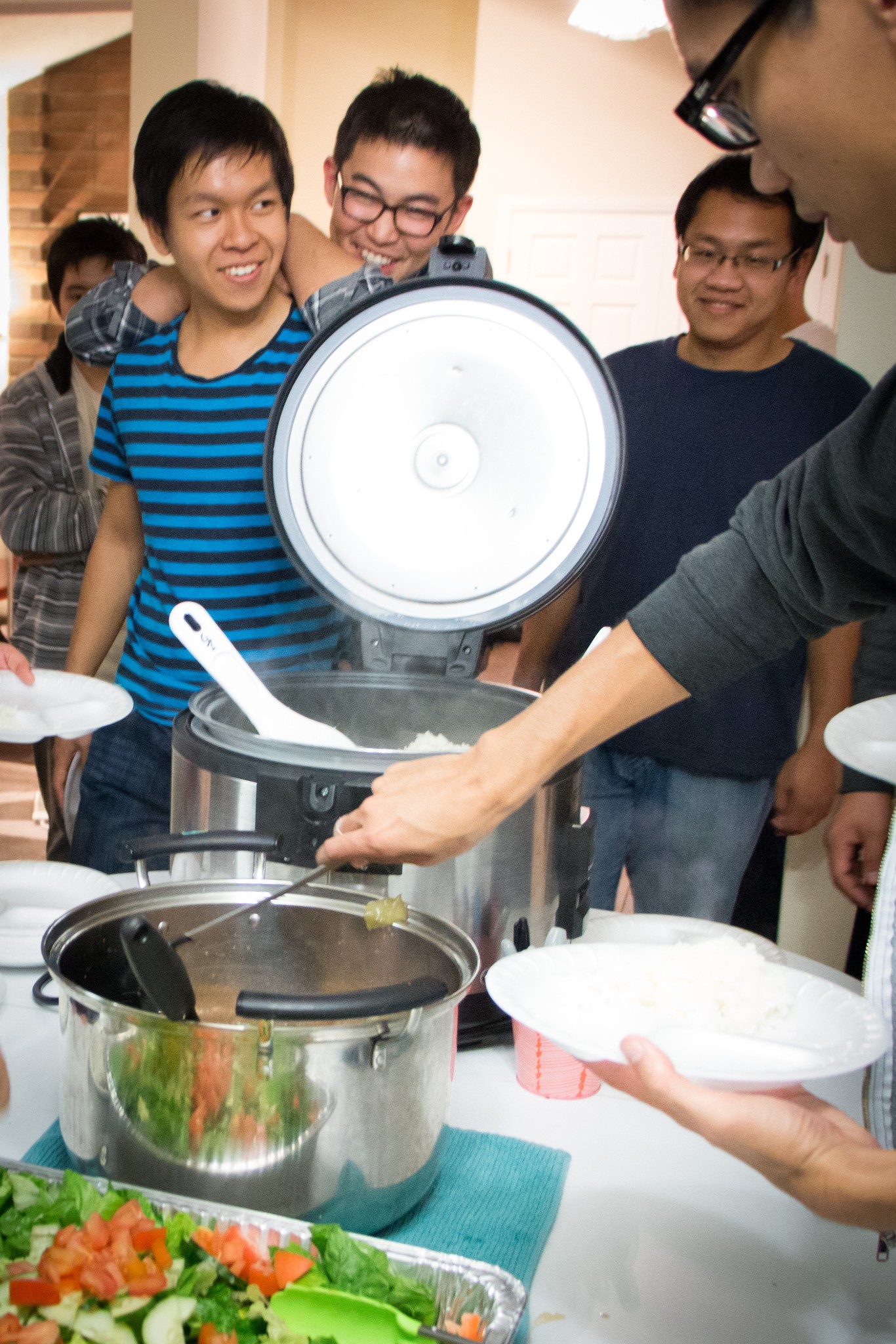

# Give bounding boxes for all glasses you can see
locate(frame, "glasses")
[674,1,779,151]
[678,234,804,278]
[336,170,458,239]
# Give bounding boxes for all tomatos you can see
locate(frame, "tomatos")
[0,1198,481,1344]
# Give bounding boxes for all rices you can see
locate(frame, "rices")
[399,730,471,752]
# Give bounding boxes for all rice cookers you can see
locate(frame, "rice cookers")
[168,234,626,1052]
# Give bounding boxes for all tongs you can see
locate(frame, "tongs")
[268,1282,485,1344]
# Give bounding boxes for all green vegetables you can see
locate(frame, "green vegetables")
[108,1018,313,1159]
[0,1168,444,1344]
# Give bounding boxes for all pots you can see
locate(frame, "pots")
[40,829,482,1236]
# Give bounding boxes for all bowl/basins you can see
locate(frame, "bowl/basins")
[0,859,124,968]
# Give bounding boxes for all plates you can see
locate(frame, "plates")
[486,940,888,1092]
[824,693,896,785]
[587,914,787,967]
[1,668,134,743]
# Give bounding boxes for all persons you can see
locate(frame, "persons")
[311,0,896,1243]
[0,70,885,977]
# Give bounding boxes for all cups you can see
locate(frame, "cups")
[512,1018,602,1098]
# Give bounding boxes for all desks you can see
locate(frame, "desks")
[0,853,896,1343]
